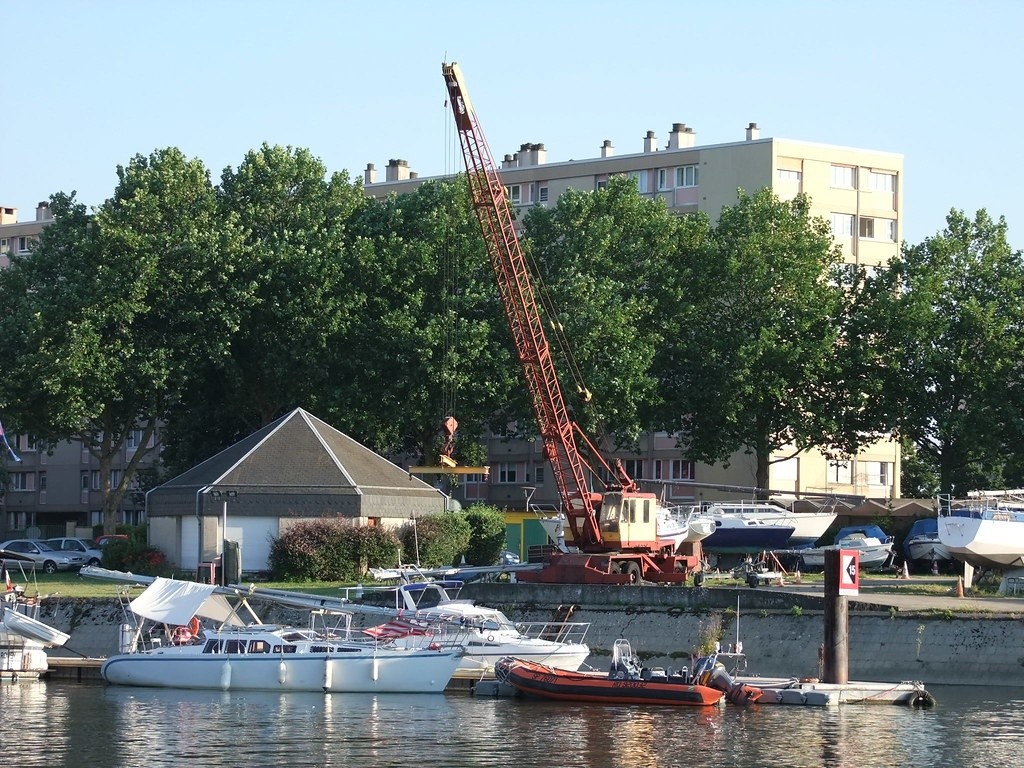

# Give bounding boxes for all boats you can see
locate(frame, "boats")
[5,606,70,648]
[492,654,762,705]
[0,560,52,682]
[530,483,1024,574]
[366,562,543,585]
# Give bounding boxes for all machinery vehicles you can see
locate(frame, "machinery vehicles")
[439,54,701,585]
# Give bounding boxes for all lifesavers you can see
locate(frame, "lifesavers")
[799,677,819,682]
[907,690,936,707]
[176,629,191,642]
[186,617,199,636]
[25,599,35,605]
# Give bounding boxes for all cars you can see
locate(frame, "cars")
[43,538,104,568]
[75,562,593,696]
[0,538,89,575]
[95,534,130,546]
